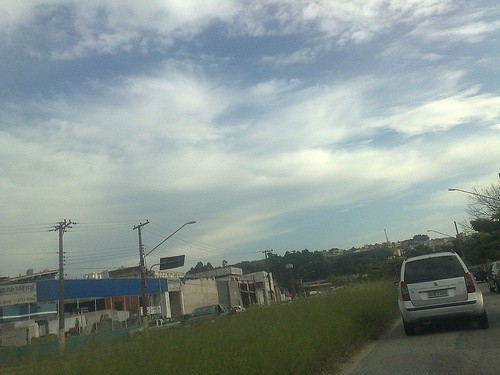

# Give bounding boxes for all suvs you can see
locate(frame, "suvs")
[397,248,488,336]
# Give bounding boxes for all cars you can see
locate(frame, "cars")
[487,260,500,294]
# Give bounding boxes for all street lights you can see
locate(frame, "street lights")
[427,229,456,239]
[448,188,500,202]
[144,220,197,258]
